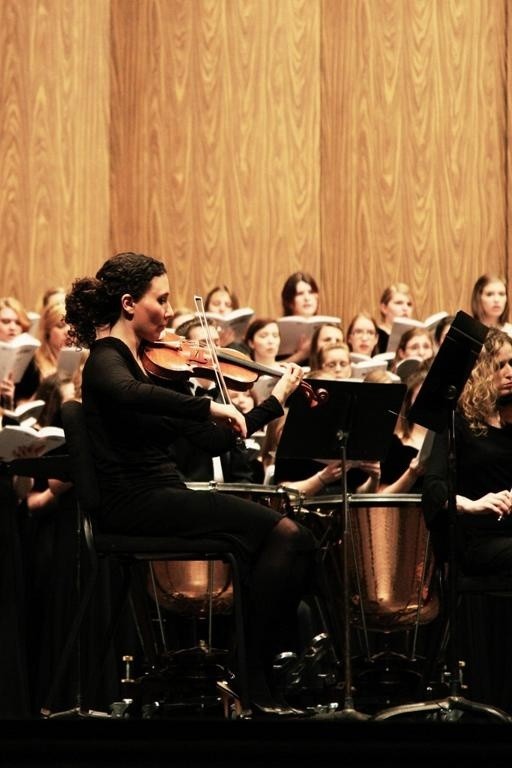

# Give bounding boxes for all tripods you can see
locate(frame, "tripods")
[298,438,371,726]
[369,416,512,726]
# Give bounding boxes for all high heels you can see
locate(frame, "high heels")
[234,687,311,717]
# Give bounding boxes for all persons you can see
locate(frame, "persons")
[3,253,510,719]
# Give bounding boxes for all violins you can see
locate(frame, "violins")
[140,331,328,409]
